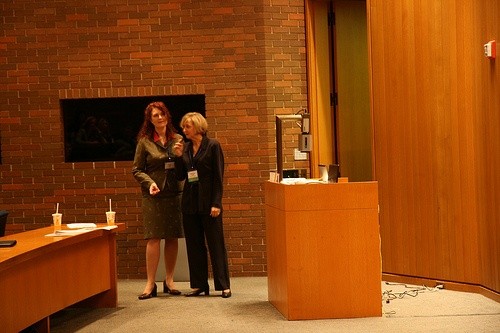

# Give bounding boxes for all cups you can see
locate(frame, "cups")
[105,211,116,225]
[51,213,62,227]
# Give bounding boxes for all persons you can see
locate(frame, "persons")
[174,112,231,298]
[131,101,185,300]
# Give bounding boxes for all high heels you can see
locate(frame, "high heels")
[164,280,181,295]
[222,289,231,297]
[184,285,209,296]
[139,283,157,298]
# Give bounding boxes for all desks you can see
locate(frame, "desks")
[0,223,126,333]
